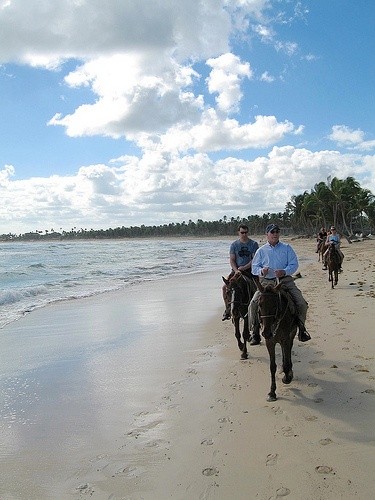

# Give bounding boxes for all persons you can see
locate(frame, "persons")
[315,228,328,253]
[247,223,312,347]
[220,225,260,322]
[321,225,344,272]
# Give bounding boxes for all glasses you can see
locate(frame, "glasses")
[240,231,248,234]
[270,231,280,234]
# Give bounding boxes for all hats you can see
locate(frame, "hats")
[266,224,280,233]
[330,226,337,230]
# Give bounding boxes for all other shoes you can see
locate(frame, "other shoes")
[249,334,261,345]
[298,333,311,341]
[222,314,230,321]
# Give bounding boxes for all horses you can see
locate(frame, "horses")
[317,236,327,262]
[252,277,299,402]
[325,243,343,289]
[222,272,259,360]
